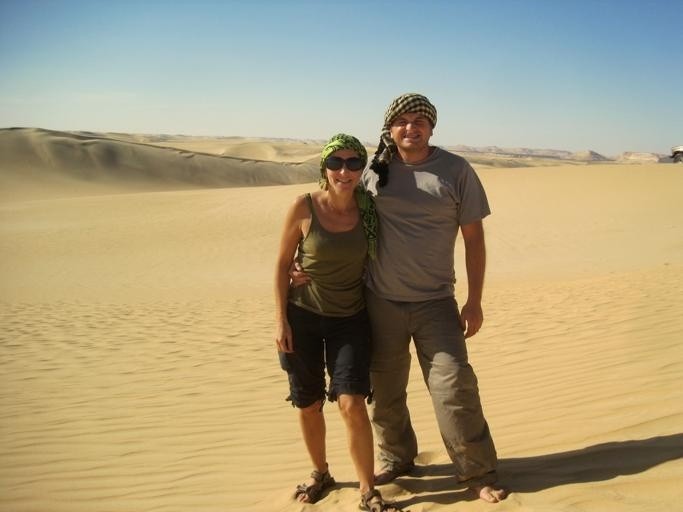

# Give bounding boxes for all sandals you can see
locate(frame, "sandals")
[358,490,401,512]
[294,463,335,504]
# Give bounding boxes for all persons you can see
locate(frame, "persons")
[271,131,401,512]
[286,90,508,502]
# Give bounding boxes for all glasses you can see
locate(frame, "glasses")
[325,157,364,171]
